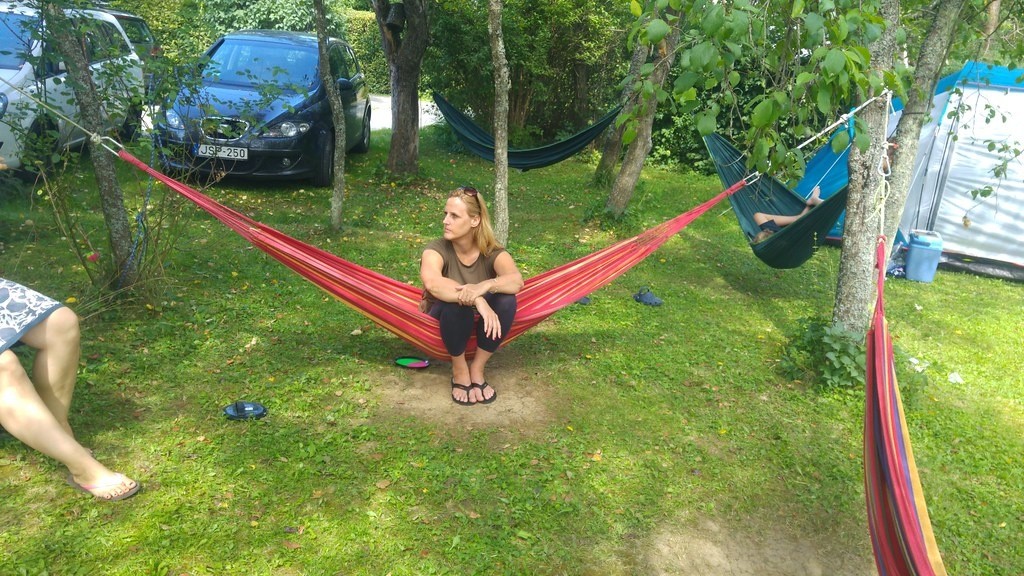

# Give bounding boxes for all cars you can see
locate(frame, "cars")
[0,8,145,169]
[153,30,372,189]
[87,7,166,88]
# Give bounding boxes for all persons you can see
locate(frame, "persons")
[0,276,140,502]
[419,187,525,407]
[752,185,824,270]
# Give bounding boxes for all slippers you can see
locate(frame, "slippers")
[633,287,663,306]
[574,296,590,305]
[451,377,478,406]
[471,381,496,404]
[69,471,141,501]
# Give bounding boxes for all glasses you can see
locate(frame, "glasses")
[456,186,482,217]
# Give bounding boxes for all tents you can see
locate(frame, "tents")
[791,60,1024,281]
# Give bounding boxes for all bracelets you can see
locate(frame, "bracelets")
[488,279,498,294]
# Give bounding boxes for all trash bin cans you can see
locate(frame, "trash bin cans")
[904,233,944,283]
